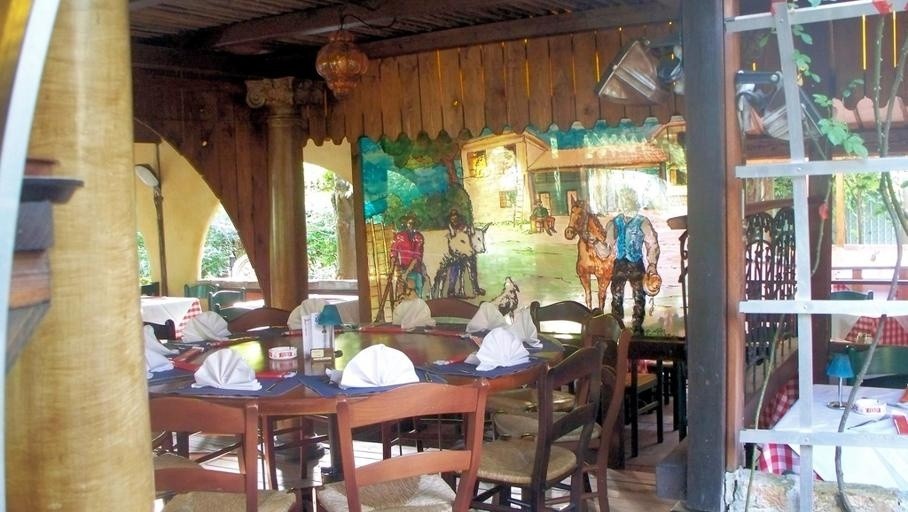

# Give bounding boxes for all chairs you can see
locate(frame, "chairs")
[830,291,874,301]
[846,345,908,389]
[536,220,544,232]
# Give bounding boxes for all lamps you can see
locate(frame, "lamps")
[594,28,836,141]
[315,0,396,104]
[827,354,855,409]
[135,164,161,195]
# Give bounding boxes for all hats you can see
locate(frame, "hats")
[642,273,662,297]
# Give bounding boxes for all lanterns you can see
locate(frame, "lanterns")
[315,30,367,98]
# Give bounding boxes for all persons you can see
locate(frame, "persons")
[590,189,661,336]
[448,209,485,296]
[530,199,557,236]
[390,211,424,298]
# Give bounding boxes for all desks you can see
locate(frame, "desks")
[769,384,907,494]
